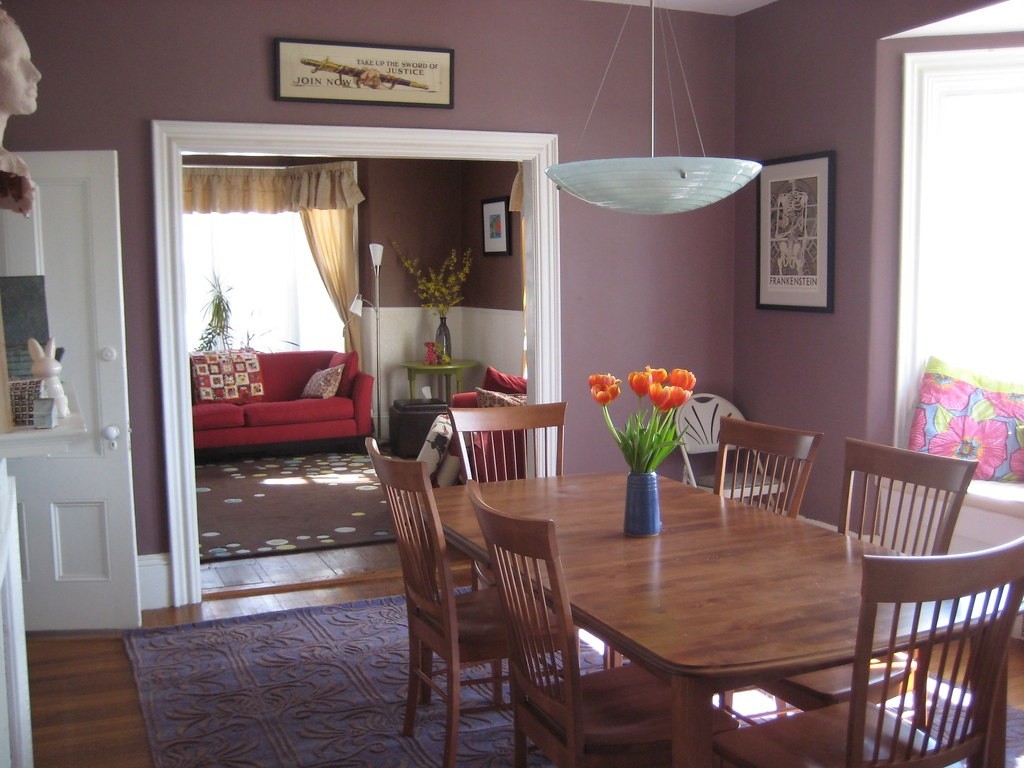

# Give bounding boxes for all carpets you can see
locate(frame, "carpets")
[197,435,434,562]
[120,578,604,767]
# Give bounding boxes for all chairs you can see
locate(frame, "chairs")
[468,489,738,768]
[675,393,787,516]
[447,403,565,483]
[714,535,1024,767]
[754,438,979,728]
[716,413,823,517]
[365,434,576,768]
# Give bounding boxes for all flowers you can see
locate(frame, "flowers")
[588,365,697,472]
[390,237,474,317]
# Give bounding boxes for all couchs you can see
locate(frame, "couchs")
[453,380,527,479]
[190,351,374,459]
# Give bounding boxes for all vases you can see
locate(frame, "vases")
[436,316,452,364]
[625,471,662,537]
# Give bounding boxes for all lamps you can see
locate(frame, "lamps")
[546,1,763,217]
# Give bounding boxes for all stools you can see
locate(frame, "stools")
[389,398,448,457]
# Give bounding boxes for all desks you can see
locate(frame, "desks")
[426,469,1023,767]
[401,361,477,401]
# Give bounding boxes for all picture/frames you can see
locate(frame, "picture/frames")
[481,195,510,257]
[273,37,455,109]
[754,149,838,311]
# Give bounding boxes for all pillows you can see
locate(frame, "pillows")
[483,365,527,395]
[475,387,528,408]
[302,363,345,400]
[327,351,358,398]
[906,357,1024,484]
